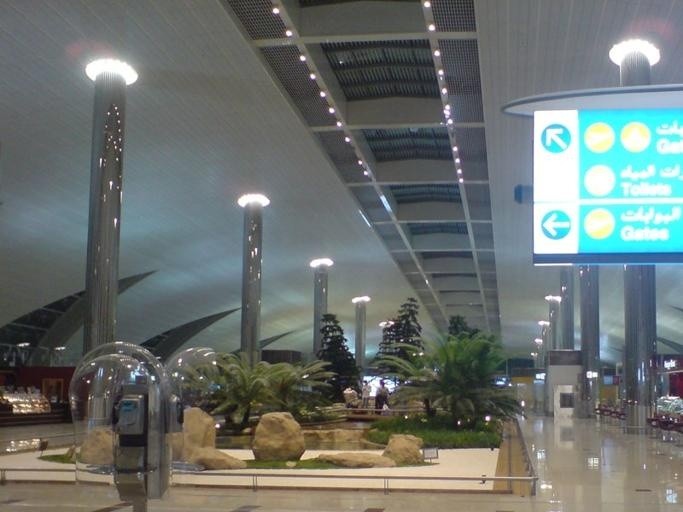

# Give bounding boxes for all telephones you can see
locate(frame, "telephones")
[111,394,145,435]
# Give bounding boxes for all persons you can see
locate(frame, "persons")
[375,380,392,411]
[360,380,371,408]
